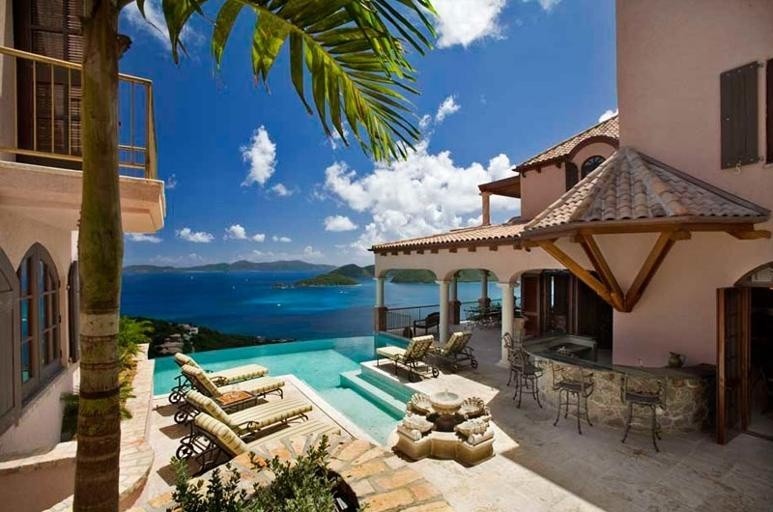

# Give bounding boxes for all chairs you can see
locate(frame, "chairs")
[426,330,479,373]
[375,333,439,382]
[168,352,341,479]
[412,312,440,337]
[502,331,668,453]
